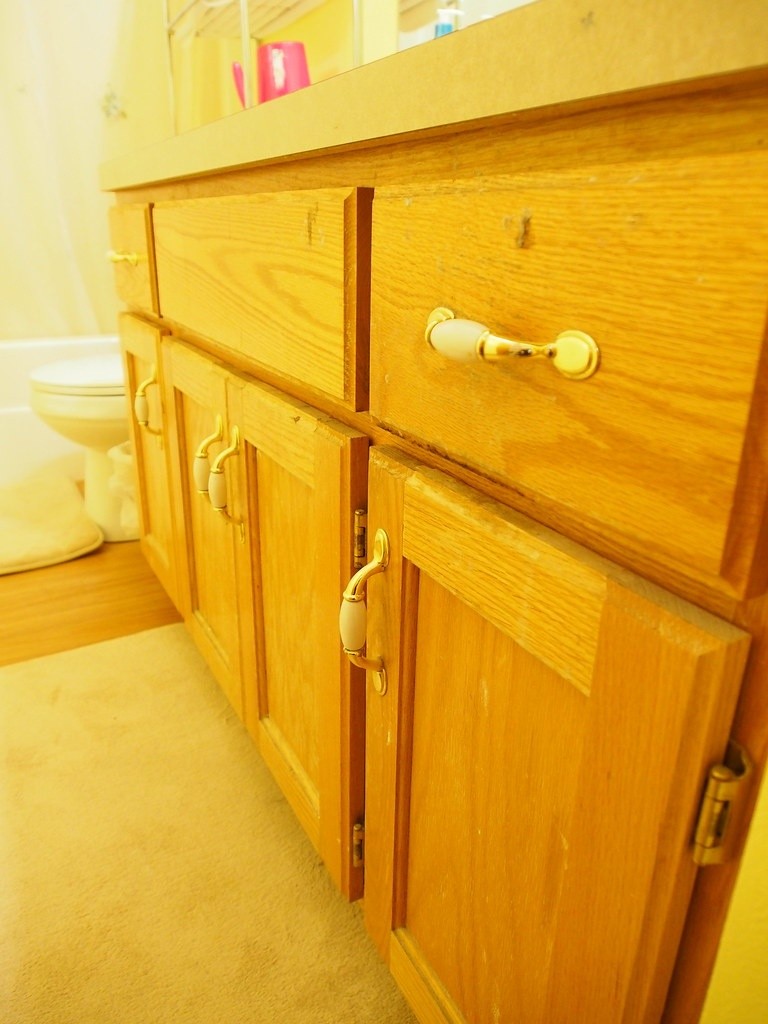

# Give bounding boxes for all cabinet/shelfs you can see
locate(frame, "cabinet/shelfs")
[149,177,364,901]
[340,85,768,1024]
[109,191,194,617]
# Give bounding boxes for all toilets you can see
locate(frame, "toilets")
[29,354,142,543]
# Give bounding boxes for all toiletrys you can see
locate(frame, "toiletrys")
[434,8,465,39]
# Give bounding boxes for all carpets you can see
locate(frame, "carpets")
[0,622,413,1024]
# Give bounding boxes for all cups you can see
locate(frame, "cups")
[233,42,311,110]
